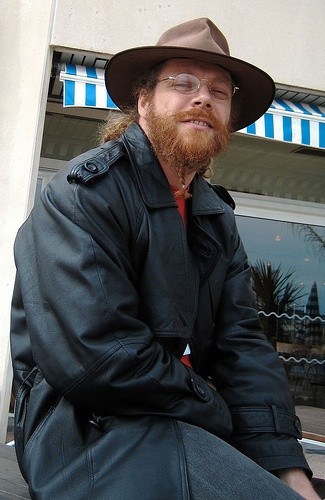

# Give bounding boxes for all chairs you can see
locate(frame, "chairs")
[291,359,325,408]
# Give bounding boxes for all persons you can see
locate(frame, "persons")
[9,17,322,500]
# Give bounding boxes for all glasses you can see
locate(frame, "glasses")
[157,72,235,101]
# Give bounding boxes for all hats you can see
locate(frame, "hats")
[105,18,276,134]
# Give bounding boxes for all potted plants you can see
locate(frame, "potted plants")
[275,319,312,353]
[248,260,309,338]
